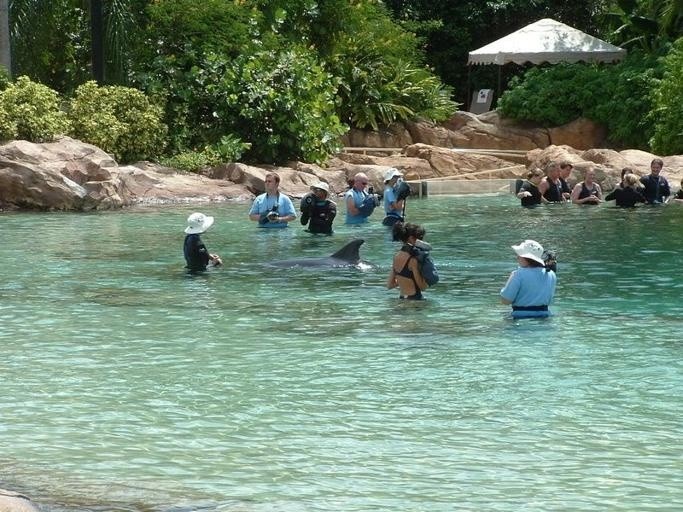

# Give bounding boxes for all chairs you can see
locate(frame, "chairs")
[469,88,493,115]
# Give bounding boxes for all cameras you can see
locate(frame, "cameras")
[267,205,278,216]
[368,186,383,206]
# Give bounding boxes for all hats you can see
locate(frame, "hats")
[310,182,330,199]
[511,239,545,267]
[383,168,403,184]
[184,212,214,235]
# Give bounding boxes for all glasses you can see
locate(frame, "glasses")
[312,188,326,195]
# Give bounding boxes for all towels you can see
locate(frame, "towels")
[476,86,491,104]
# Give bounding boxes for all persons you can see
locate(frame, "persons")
[381,167,407,226]
[636,158,670,206]
[300,182,336,233]
[612,166,646,192]
[247,172,296,228]
[387,223,427,300]
[183,212,222,273]
[558,160,572,201]
[604,173,648,209]
[569,165,602,205]
[343,172,370,224]
[673,178,682,202]
[536,160,567,203]
[516,166,548,207]
[498,239,556,320]
